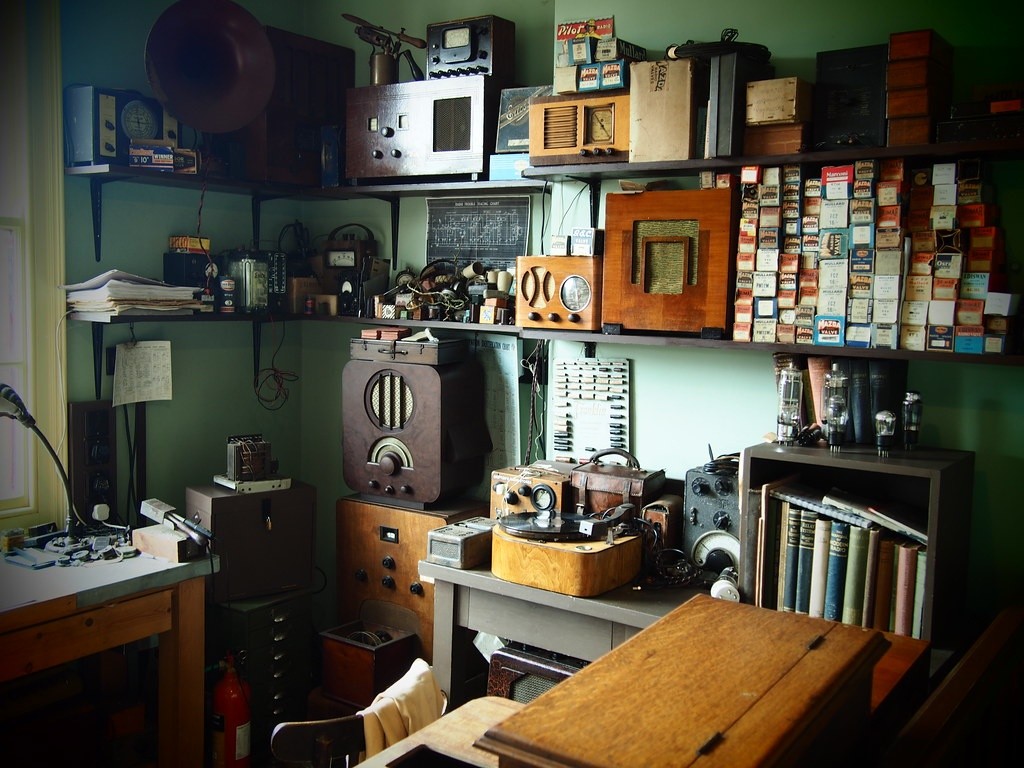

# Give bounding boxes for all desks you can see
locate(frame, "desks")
[0,530,221,768]
[349,441,977,768]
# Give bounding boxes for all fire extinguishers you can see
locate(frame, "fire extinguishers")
[204,642,253,768]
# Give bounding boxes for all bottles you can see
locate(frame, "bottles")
[306,297,314,313]
[220,275,238,317]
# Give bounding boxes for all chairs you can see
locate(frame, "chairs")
[268,657,448,768]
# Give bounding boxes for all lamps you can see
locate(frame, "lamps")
[0,383,93,555]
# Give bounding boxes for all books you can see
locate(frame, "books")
[742,473,928,639]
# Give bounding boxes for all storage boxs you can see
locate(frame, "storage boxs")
[129,138,198,174]
[169,235,211,255]
[552,28,1024,356]
[319,620,416,710]
[348,337,467,365]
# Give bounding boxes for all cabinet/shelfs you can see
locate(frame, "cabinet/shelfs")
[208,586,316,753]
[63,136,1024,400]
